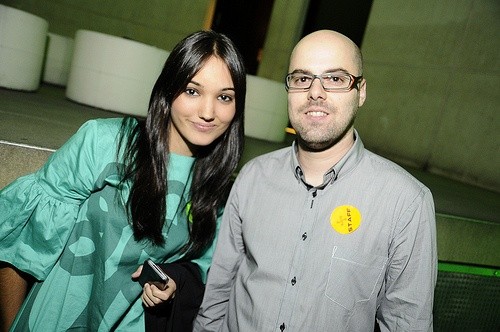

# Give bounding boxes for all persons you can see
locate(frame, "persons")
[192,29,438,332]
[0,31,247,332]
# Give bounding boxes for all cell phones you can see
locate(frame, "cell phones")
[138,259,169,291]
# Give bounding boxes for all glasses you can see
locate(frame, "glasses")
[285,72,362,92]
